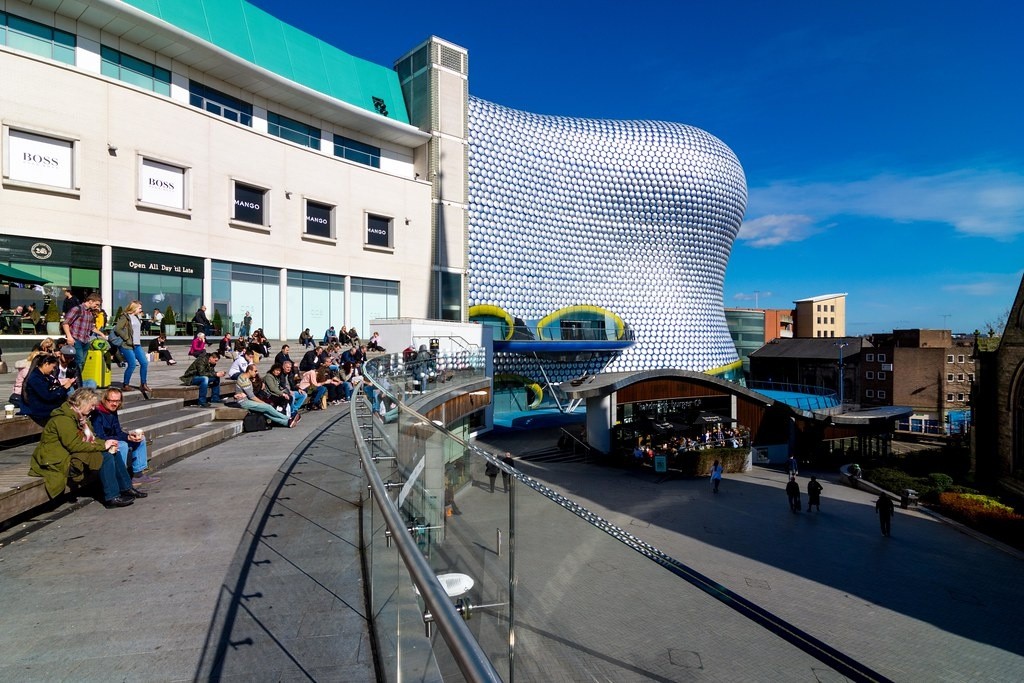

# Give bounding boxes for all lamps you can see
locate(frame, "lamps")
[285,190,293,196]
[405,216,412,223]
[107,142,119,151]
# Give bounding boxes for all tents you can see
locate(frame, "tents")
[0,263,54,286]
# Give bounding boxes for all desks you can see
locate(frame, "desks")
[141,318,153,335]
[0,315,22,334]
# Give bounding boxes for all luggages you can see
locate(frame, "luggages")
[80,349,113,387]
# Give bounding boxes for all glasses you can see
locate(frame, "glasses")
[105,398,121,403]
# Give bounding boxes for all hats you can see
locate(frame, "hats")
[853,464,859,469]
[419,345,427,351]
[57,338,77,359]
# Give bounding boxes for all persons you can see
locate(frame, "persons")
[473,320,479,324]
[217,327,385,427]
[179,352,226,408]
[846,447,853,460]
[785,477,801,514]
[402,345,431,395]
[444,477,462,516]
[240,311,252,336]
[709,460,723,495]
[28,387,161,508]
[875,491,894,537]
[0,290,177,427]
[633,423,750,469]
[191,305,211,336]
[623,321,630,340]
[786,455,797,482]
[807,475,823,512]
[485,453,515,494]
[363,378,399,424]
[848,463,862,488]
[188,332,207,358]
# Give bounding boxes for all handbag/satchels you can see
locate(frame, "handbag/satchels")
[243,413,267,431]
[89,337,111,351]
[797,501,802,511]
[108,325,126,347]
[9,393,22,406]
[322,396,328,410]
[146,351,160,362]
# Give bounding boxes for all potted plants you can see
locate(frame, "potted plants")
[161,305,176,336]
[46,299,62,335]
[212,310,223,335]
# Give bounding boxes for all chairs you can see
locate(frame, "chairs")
[21,317,36,335]
[176,314,188,335]
[192,322,198,337]
[150,323,161,335]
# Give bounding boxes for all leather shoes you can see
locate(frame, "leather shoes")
[122,386,135,391]
[140,383,152,392]
[121,488,147,498]
[105,495,135,508]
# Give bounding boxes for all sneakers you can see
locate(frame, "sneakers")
[132,474,161,486]
[288,412,301,428]
[332,398,351,407]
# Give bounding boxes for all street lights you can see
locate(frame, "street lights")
[832,342,851,404]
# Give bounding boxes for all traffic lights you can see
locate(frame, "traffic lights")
[945,414,951,424]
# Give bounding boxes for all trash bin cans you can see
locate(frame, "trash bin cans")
[901,489,919,510]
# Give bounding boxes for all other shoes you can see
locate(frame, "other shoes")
[198,402,210,408]
[168,361,176,365]
[713,488,717,494]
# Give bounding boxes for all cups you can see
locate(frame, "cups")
[135,429,143,439]
[4,404,15,418]
